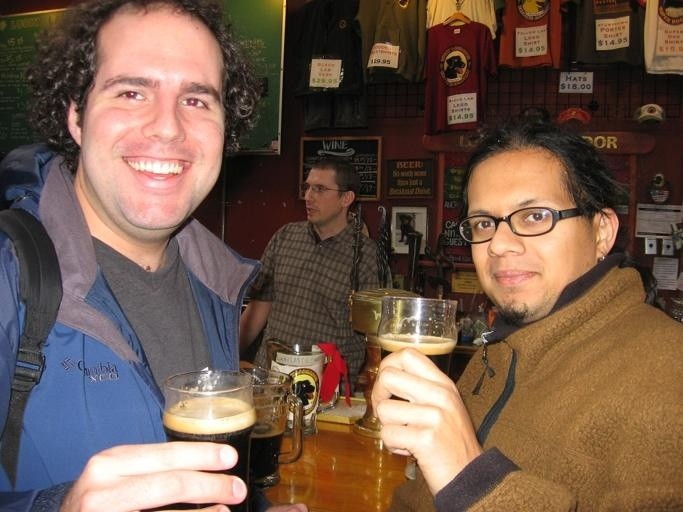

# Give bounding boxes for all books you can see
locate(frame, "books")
[314,396,368,427]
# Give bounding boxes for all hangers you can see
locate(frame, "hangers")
[439,0,473,26]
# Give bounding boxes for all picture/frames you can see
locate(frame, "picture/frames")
[299,137,435,204]
[390,207,429,256]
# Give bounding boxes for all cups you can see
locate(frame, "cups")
[275,346,322,435]
[245,371,302,485]
[378,295,459,401]
[162,371,258,512]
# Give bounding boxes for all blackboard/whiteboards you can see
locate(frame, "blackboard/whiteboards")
[299,136,382,202]
[0,0,287,157]
[386,158,434,199]
[442,218,472,247]
[443,166,467,209]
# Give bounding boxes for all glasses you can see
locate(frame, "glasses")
[458,206,582,243]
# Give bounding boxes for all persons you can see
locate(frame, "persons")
[238,153,390,391]
[1,2,308,512]
[369,113,683,511]
